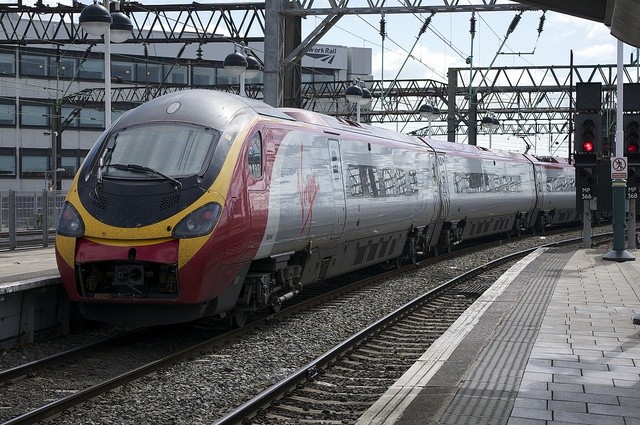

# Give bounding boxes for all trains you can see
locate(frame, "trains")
[54,89,629,331]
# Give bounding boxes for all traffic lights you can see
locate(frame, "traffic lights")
[582,119,596,155]
[623,113,639,158]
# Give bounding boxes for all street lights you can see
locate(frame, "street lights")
[79,0,134,130]
[345,76,372,123]
[481,109,500,149]
[419,98,441,141]
[222,40,260,98]
